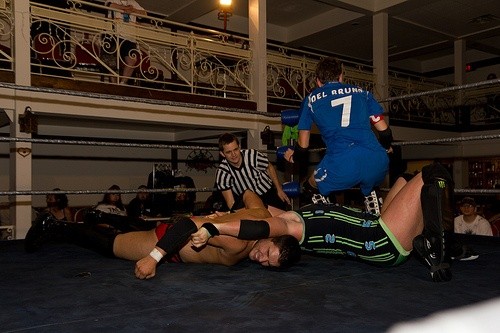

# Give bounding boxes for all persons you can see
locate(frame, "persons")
[282,57,393,216]
[63,189,302,279]
[191,172,480,284]
[105,0,148,85]
[215,134,291,213]
[454,197,493,236]
[39,187,72,222]
[127,185,162,227]
[162,167,197,217]
[93,186,127,228]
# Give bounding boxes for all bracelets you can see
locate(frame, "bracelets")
[201,223,219,238]
[150,248,163,261]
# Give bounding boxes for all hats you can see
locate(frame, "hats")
[460,197,476,206]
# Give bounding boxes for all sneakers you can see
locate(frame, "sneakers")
[413,235,452,281]
[74,208,105,224]
[23,213,56,250]
[446,249,480,261]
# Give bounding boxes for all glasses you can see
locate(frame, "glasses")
[461,204,474,208]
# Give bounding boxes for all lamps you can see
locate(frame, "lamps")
[218,0,232,33]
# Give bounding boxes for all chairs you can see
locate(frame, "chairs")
[32,34,164,89]
